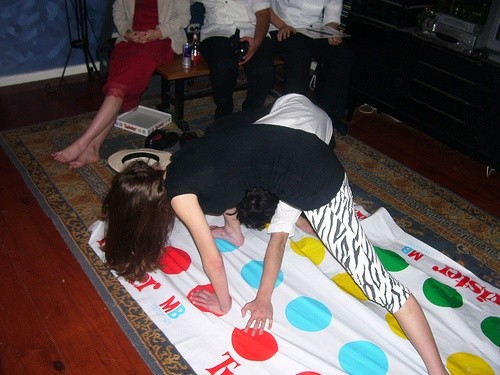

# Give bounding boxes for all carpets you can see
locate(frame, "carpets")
[0,82,500,374]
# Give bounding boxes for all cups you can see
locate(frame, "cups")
[232,41,248,61]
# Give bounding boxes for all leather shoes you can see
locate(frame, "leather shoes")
[145,129,180,150]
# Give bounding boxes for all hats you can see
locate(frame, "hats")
[108,148,171,173]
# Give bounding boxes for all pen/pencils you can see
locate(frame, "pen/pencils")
[306,29,333,35]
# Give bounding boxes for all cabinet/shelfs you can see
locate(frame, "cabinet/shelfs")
[343,0,500,170]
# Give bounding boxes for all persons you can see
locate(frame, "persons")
[189,0,273,122]
[270,0,343,137]
[226,92,335,338]
[99,122,448,375]
[52,0,192,171]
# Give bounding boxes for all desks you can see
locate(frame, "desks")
[157,53,283,121]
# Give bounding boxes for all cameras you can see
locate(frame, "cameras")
[235,40,249,60]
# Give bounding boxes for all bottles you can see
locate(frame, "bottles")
[191,33,201,62]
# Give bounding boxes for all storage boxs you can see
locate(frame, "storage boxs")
[114,104,173,137]
